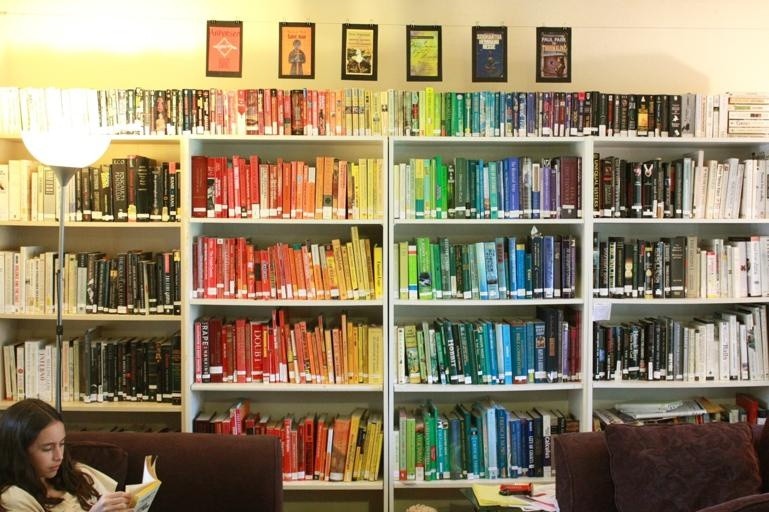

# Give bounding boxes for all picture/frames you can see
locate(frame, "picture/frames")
[205,20,243,78]
[341,24,379,81]
[405,24,443,81]
[279,22,316,79]
[471,25,509,84]
[535,26,572,84]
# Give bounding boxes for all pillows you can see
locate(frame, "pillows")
[605,423,731,509]
[69,439,130,489]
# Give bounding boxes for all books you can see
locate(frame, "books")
[126,454,162,512]
[193,308,383,384]
[1,245,181,316]
[2,327,181,406]
[592,303,769,434]
[594,151,769,300]
[0,87,768,137]
[472,485,527,507]
[393,157,583,219]
[391,398,581,480]
[394,306,582,385]
[194,400,383,482]
[191,154,382,220]
[193,225,384,301]
[393,232,576,299]
[1,155,181,223]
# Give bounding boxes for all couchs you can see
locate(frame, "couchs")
[59,432,281,512]
[554,425,768,512]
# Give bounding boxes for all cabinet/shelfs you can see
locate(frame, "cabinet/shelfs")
[590,140,768,429]
[184,133,389,510]
[390,137,591,512]
[43,138,181,416]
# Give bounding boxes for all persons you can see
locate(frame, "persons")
[1,399,132,512]
[289,39,306,75]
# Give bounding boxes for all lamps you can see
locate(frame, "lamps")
[21,125,114,411]
